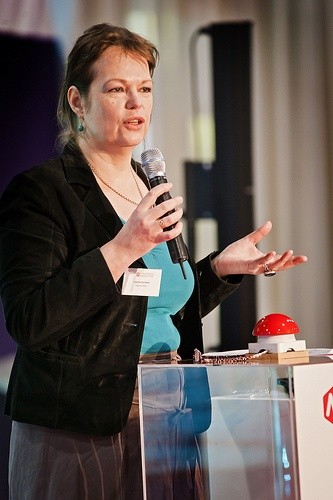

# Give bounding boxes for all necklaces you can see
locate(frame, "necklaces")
[89,163,143,205]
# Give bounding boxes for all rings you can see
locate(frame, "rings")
[159,219,165,228]
[264,264,276,277]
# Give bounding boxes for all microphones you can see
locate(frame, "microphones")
[142,148,189,263]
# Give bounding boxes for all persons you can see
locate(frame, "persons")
[0,24,307,500]
